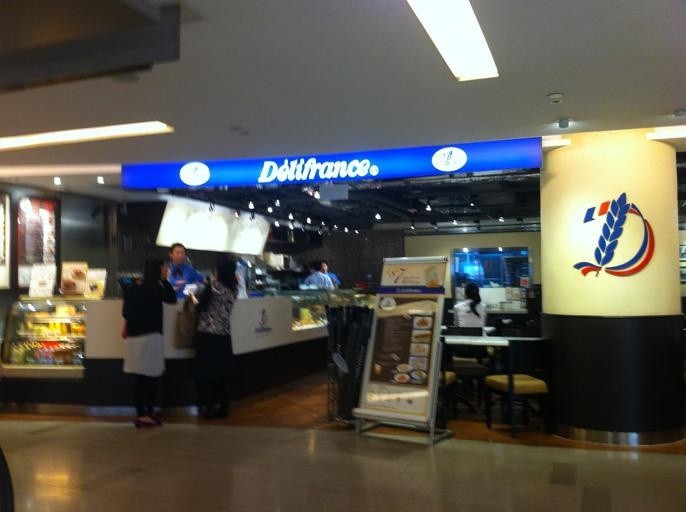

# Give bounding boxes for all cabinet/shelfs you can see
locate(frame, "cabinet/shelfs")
[0,295,86,381]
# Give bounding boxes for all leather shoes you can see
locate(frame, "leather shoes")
[134,410,164,428]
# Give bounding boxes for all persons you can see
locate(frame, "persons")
[454,283,487,325]
[123,243,238,429]
[304,257,340,290]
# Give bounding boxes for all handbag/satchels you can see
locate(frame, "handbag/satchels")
[173,294,201,349]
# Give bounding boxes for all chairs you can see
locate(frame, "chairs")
[438,336,554,438]
